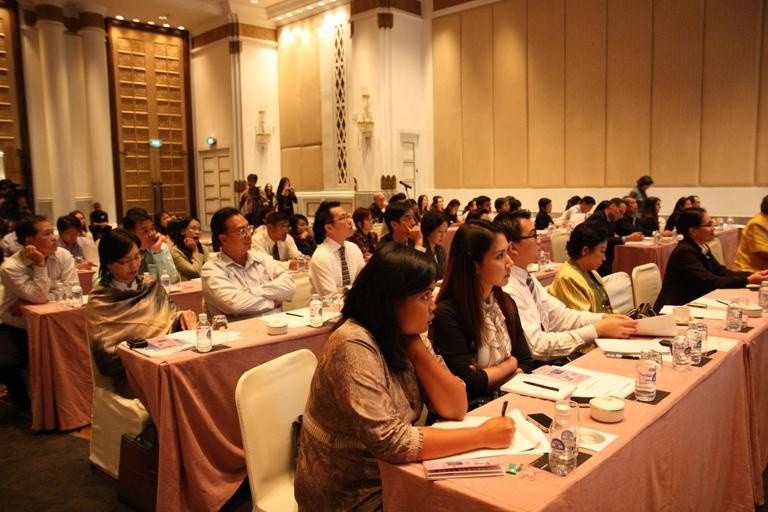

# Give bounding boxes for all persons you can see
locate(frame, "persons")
[630,176,654,205]
[663,197,693,234]
[690,195,700,209]
[655,208,768,318]
[91,202,108,239]
[277,177,298,213]
[462,198,479,222]
[291,215,317,256]
[386,193,406,209]
[550,219,617,355]
[565,197,582,210]
[444,199,460,224]
[491,197,511,222]
[508,196,522,211]
[87,227,198,398]
[1,208,34,259]
[237,174,269,227]
[309,202,366,306]
[534,198,556,229]
[294,245,517,512]
[377,202,427,253]
[170,218,209,280]
[491,210,637,367]
[55,215,93,270]
[466,197,490,222]
[637,196,662,234]
[732,193,768,273]
[430,196,446,214]
[0,214,84,421]
[349,207,378,263]
[586,198,645,275]
[123,207,181,285]
[70,211,99,266]
[251,212,301,263]
[368,192,388,223]
[418,195,430,218]
[615,196,674,237]
[264,184,279,214]
[428,220,532,411]
[201,206,296,317]
[559,196,596,228]
[157,211,175,232]
[421,210,447,281]
[404,199,421,222]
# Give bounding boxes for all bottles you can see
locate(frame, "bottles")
[159,269,170,296]
[537,250,548,272]
[654,230,662,248]
[67,281,83,309]
[549,404,577,476]
[54,282,66,311]
[758,281,768,313]
[309,294,323,328]
[547,222,553,236]
[672,226,677,241]
[685,321,702,365]
[719,218,724,231]
[634,350,657,402]
[726,295,743,332]
[195,313,212,353]
[726,215,734,230]
[141,273,150,290]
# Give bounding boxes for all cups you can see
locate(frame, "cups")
[171,272,182,294]
[639,347,663,380]
[265,322,288,336]
[738,297,749,331]
[671,306,691,325]
[588,394,627,424]
[552,399,579,447]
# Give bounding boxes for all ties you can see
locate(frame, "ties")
[339,247,351,287]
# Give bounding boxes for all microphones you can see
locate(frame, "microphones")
[400,181,411,190]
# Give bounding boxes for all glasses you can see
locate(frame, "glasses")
[114,255,144,266]
[227,225,255,238]
[697,222,714,227]
[519,230,537,240]
[326,214,352,224]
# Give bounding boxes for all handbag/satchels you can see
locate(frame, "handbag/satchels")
[627,303,656,321]
[116,427,159,512]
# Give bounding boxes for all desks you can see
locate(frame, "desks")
[115,307,341,512]
[613,224,746,285]
[528,263,564,288]
[376,289,767,511]
[19,280,202,430]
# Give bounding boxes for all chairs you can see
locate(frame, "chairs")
[599,271,634,314]
[550,233,573,263]
[281,272,312,311]
[84,321,150,479]
[631,263,661,309]
[234,348,318,512]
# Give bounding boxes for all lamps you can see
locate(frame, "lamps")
[357,94,374,141]
[254,111,272,147]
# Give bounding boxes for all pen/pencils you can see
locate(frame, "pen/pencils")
[607,354,639,360]
[285,313,304,317]
[524,381,559,391]
[501,401,509,416]
[715,299,729,308]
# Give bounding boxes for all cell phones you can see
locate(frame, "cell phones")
[570,396,596,408]
[127,337,147,349]
[527,413,562,432]
[687,302,708,308]
